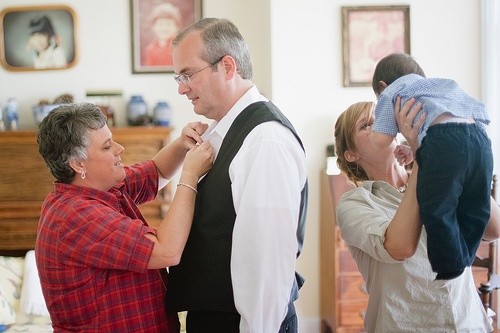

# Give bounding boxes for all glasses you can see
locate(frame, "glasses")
[174,54,229,85]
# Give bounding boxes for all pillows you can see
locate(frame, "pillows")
[20,250,50,316]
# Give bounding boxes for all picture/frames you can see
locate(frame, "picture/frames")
[130,0,204,74]
[0,3,80,72]
[341,4,411,88]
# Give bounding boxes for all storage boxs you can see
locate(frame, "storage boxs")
[31,104,71,126]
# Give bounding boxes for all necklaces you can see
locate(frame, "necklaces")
[395,182,409,193]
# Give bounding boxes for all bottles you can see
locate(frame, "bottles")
[6,97,19,129]
[126,96,148,125]
[153,102,171,126]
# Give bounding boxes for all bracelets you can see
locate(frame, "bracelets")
[176,183,199,196]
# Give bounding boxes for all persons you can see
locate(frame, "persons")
[144,3,182,65]
[333,101,500,333]
[368,52,494,288]
[33,102,215,333]
[164,17,310,333]
[27,14,68,67]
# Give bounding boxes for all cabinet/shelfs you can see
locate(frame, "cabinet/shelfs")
[0,126,173,256]
[319,167,500,333]
